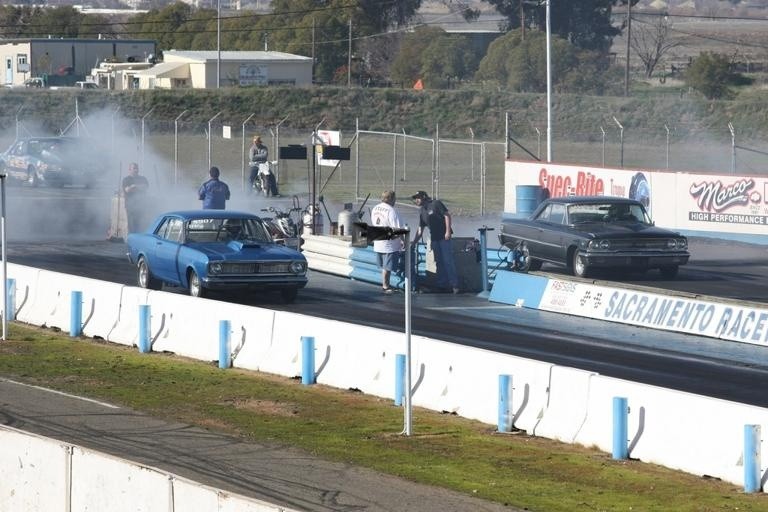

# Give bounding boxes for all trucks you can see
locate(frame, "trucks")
[21,77,74,90]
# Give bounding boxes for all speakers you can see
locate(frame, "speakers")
[280,146,307,159]
[323,147,351,159]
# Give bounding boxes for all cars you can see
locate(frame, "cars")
[0,134,98,191]
[496,193,692,279]
[124,209,309,298]
[76,81,100,89]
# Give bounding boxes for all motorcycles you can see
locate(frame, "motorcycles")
[259,205,307,252]
[247,160,281,198]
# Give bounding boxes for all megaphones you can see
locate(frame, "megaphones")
[350,222,392,248]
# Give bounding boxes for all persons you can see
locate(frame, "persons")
[197,167,231,230]
[370,191,405,292]
[246,136,281,199]
[410,191,461,294]
[122,162,148,234]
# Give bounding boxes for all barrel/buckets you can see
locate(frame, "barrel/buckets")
[516,185,542,217]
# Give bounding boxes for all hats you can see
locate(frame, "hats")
[412,191,427,199]
